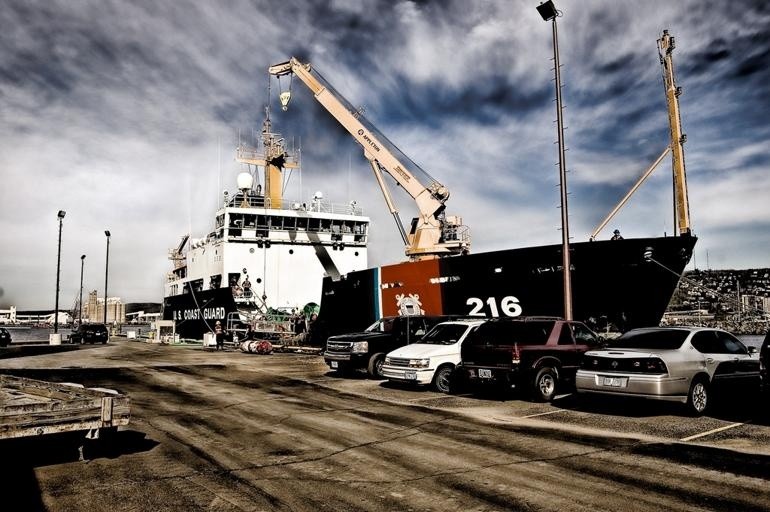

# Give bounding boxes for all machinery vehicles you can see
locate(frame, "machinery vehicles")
[269,55,471,262]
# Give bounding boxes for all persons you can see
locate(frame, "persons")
[242,278,251,298]
[214,321,225,350]
[611,229,624,240]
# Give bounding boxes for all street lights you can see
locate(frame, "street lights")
[103,230,111,324]
[79,255,86,324]
[534,0,573,323]
[55,209,66,333]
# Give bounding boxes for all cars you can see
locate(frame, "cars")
[575,324,761,418]
[381,320,489,393]
[323,315,486,380]
[68,322,109,345]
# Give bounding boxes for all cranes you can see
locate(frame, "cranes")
[586,25,694,244]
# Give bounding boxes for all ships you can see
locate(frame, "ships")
[159,104,699,366]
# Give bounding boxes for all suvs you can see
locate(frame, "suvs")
[460,314,608,403]
